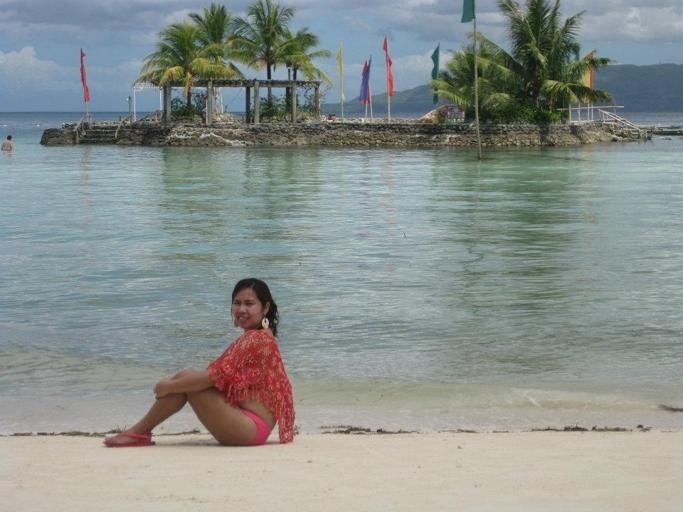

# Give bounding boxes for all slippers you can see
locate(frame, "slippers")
[104,432,155,446]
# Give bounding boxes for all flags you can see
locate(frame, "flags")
[430,43,440,104]
[461,0,475,24]
[335,44,343,101]
[358,55,371,99]
[383,35,395,97]
[572,51,593,100]
[79,49,90,103]
[359,58,370,104]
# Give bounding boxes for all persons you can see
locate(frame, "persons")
[1,135,12,151]
[102,277,295,447]
